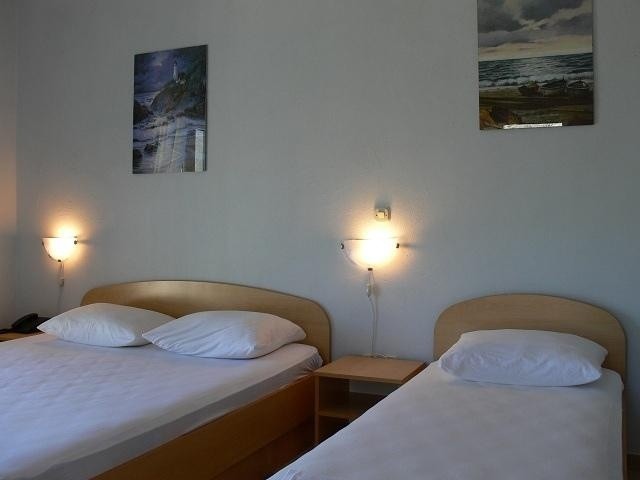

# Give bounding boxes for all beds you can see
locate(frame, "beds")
[265,293,627,480]
[0,279,330,480]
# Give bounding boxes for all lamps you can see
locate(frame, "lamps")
[42,234,81,317]
[341,235,400,357]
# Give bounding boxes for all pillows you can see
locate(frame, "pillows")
[438,329,609,387]
[36,303,176,347]
[142,310,306,360]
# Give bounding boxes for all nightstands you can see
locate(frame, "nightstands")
[314,355,426,447]
[0,333,40,341]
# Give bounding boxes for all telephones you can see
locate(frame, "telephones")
[0,313,50,334]
